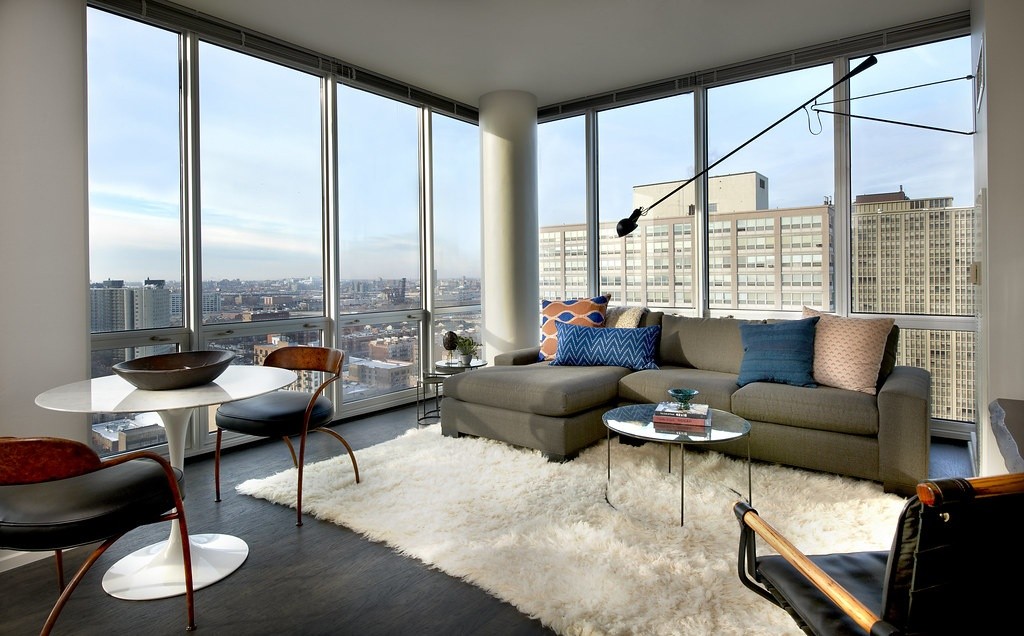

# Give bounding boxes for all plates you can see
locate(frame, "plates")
[111,348,238,391]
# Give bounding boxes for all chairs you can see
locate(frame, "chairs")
[0,436,198,635]
[733,473,1024,636]
[215,346,360,525]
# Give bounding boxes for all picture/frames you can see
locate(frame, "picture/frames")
[976,32,984,113]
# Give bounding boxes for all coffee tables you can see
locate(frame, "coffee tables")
[602,403,753,527]
[435,359,487,374]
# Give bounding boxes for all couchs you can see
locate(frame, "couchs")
[441,306,931,500]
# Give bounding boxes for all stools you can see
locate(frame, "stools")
[417,372,451,426]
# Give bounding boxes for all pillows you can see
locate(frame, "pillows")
[736,315,819,389]
[550,319,661,371]
[539,293,611,360]
[801,304,895,394]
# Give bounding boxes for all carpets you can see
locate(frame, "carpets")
[237,422,909,636]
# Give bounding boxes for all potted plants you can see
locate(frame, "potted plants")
[456,336,483,365]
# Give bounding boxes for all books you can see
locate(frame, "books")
[653,401,713,426]
[652,423,709,436]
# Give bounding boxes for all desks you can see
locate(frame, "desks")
[34,365,298,600]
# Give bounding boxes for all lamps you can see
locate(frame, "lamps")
[616,53,973,238]
